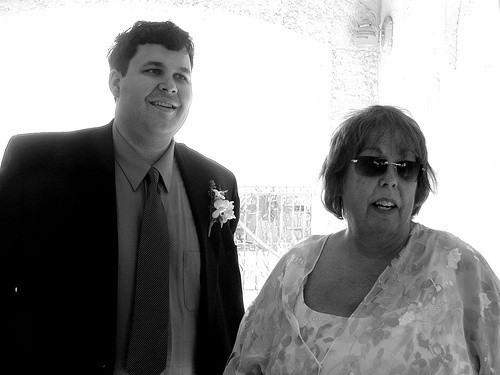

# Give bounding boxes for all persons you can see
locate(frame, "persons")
[0,21,248,375]
[222,105,500,375]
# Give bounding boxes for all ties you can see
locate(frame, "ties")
[125,167,171,375]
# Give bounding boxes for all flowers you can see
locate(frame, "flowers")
[205,178,238,238]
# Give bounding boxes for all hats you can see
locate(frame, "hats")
[350,156,425,183]
